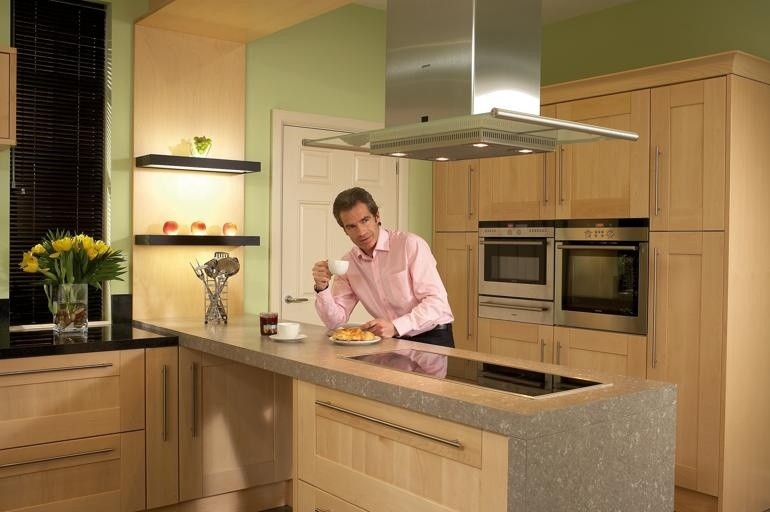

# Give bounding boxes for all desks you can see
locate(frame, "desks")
[127,308,680,511]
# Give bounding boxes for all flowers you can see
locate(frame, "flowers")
[17,226,130,327]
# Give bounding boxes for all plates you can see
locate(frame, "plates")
[328,336,383,346]
[270,335,307,342]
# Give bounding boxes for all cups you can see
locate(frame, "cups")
[259,312,278,336]
[326,259,350,277]
[276,322,300,338]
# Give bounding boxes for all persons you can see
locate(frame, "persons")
[312,187,454,348]
[369,349,448,378]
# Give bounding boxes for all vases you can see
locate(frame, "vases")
[52,282,91,345]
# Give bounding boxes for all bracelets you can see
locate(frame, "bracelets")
[314,286,327,293]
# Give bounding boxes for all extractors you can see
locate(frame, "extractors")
[298,1,645,173]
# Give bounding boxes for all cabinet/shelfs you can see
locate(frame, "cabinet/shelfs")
[144,347,293,511]
[477,317,647,380]
[1,46,19,152]
[479,65,651,221]
[432,158,479,233]
[132,151,262,248]
[651,49,770,232]
[648,231,770,511]
[431,233,478,351]
[1,320,178,511]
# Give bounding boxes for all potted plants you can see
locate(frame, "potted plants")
[190,135,213,158]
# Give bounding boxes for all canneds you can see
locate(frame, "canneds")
[261,313,278,336]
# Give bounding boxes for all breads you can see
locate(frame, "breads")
[332,326,375,340]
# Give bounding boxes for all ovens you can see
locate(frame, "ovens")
[477,219,649,334]
